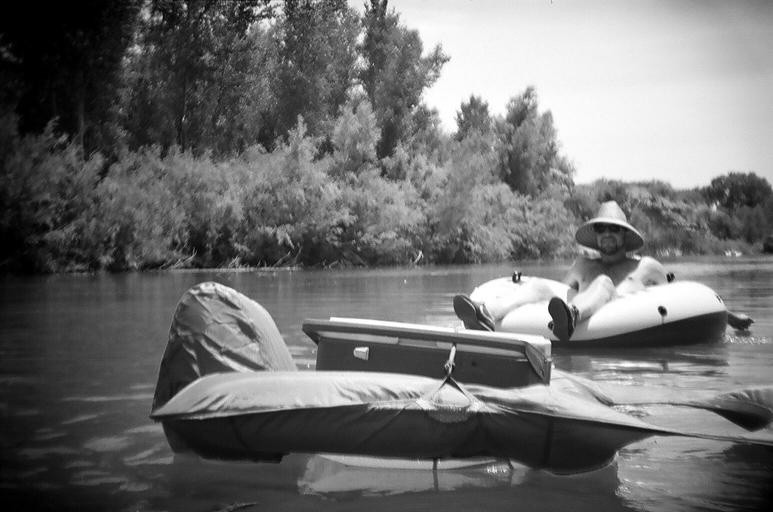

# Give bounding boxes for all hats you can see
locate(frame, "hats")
[572,198,645,254]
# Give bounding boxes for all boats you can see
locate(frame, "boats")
[465,273,727,353]
[144,276,773,497]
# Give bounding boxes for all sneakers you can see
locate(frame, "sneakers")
[546,295,576,343]
[451,294,497,334]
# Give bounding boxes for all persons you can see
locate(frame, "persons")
[453,195,669,341]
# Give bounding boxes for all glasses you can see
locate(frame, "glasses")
[589,221,626,235]
